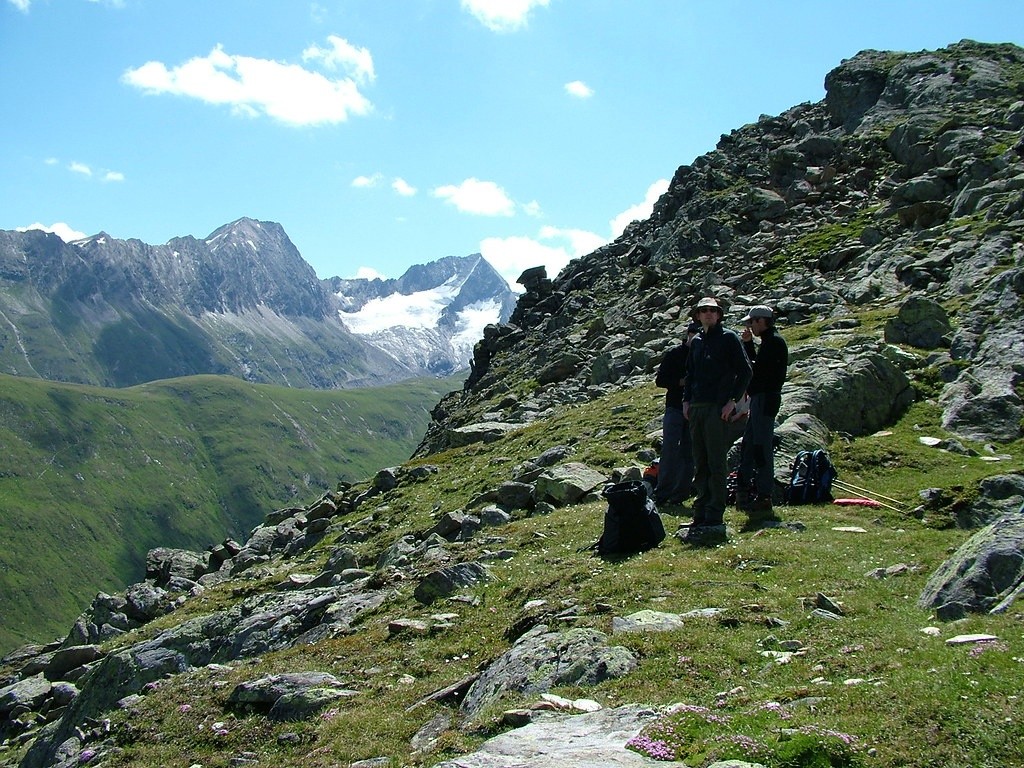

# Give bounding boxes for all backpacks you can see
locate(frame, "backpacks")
[784,449,839,505]
[642,458,661,491]
[589,480,666,554]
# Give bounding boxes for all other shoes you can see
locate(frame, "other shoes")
[735,493,749,505]
[666,489,690,504]
[678,520,703,530]
[747,494,772,510]
[697,520,722,528]
[654,482,673,497]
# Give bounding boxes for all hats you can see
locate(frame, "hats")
[741,305,774,322]
[691,298,723,327]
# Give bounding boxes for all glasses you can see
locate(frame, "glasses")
[698,308,718,313]
[749,318,758,324]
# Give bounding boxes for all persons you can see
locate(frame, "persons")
[654,322,701,507]
[679,297,754,529]
[737,305,788,510]
